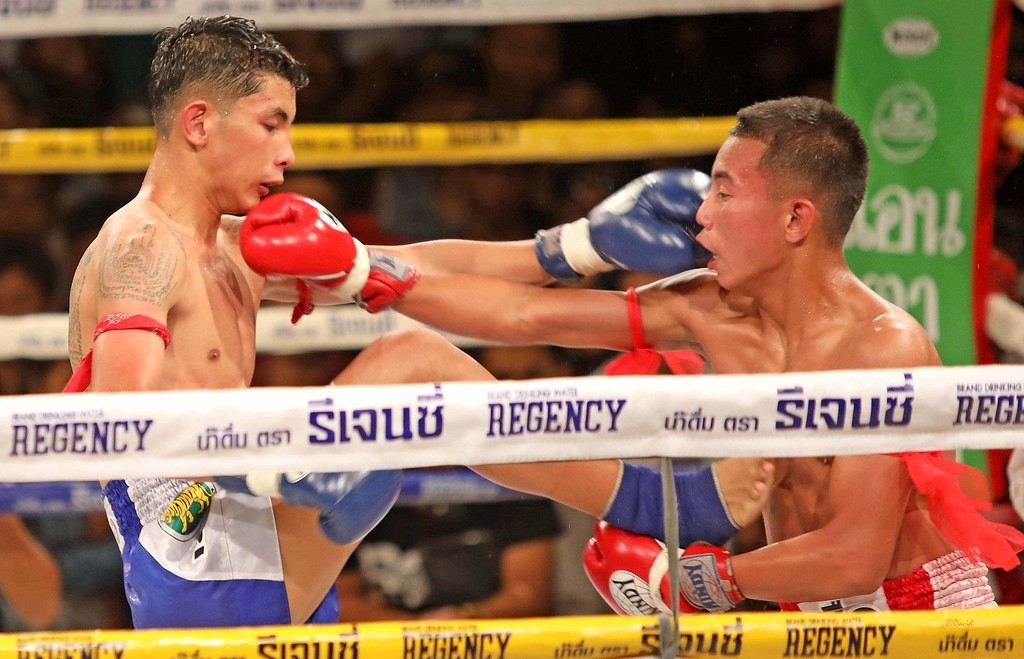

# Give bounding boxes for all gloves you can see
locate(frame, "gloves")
[239,192,422,315]
[535,170,710,285]
[584,521,744,617]
[214,469,403,546]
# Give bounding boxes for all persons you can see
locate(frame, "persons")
[0,0,1024,631]
